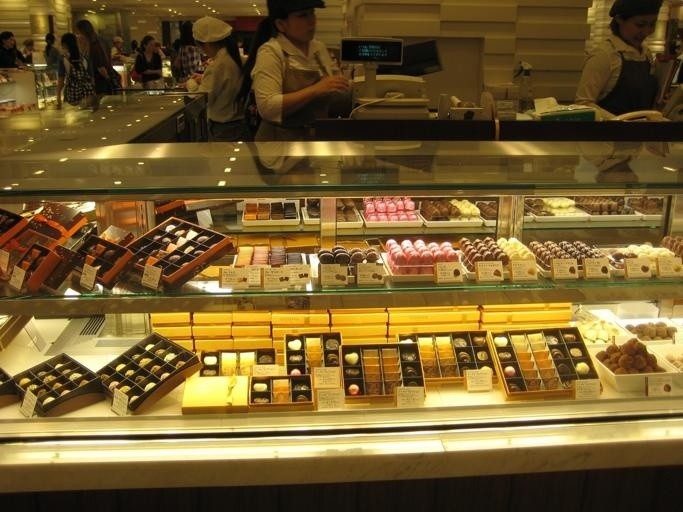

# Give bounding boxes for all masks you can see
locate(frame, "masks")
[287,28,313,46]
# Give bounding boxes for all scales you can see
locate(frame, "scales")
[338,37,429,121]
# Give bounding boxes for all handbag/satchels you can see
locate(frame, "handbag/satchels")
[130,68,144,81]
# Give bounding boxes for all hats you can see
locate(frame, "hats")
[192,17,233,44]
[267,0,325,19]
[609,0,663,18]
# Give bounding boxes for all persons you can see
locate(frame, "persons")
[575,0,683,122]
[178,14,252,141]
[571,139,644,187]
[0,19,243,113]
[250,1,355,142]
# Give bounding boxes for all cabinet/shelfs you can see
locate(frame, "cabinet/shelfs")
[1,188,682,443]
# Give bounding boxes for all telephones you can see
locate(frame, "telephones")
[610,111,672,122]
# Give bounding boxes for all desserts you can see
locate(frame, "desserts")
[238,197,668,224]
[0,215,683,416]
